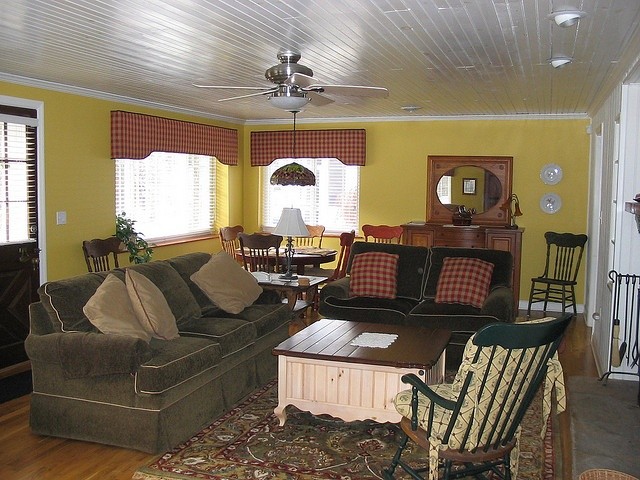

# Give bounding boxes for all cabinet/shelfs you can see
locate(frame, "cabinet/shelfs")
[482,229,524,322]
[394,228,431,247]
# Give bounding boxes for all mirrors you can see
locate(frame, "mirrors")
[428,157,515,227]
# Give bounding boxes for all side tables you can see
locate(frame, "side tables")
[250,271,328,328]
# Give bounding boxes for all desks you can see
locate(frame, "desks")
[234,247,338,274]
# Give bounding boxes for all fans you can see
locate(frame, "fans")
[188,49,393,115]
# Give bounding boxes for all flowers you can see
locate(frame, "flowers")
[112,214,158,266]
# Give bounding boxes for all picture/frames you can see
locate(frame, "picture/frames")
[462,177,478,195]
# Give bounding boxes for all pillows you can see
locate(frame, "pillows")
[79,275,154,341]
[433,256,496,308]
[189,254,269,313]
[123,270,186,341]
[342,252,401,300]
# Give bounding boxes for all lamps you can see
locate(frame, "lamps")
[542,49,578,76]
[271,208,310,280]
[270,108,315,187]
[543,6,586,30]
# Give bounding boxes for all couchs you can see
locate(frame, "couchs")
[316,241,514,367]
[23,250,298,453]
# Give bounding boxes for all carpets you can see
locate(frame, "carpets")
[130,288,561,479]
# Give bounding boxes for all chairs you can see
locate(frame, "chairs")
[525,231,587,319]
[360,222,407,245]
[383,320,579,479]
[293,225,326,251]
[238,234,280,278]
[330,228,355,283]
[217,225,243,262]
[82,237,121,273]
